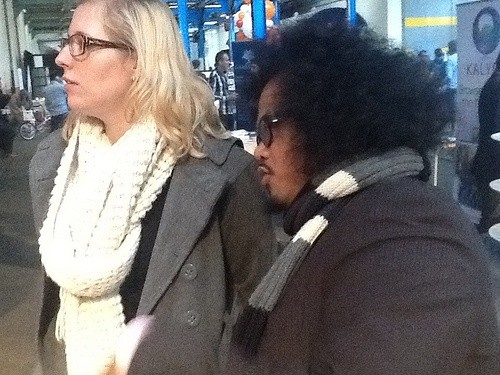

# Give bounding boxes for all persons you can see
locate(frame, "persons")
[29,1,277,374]
[221,8,500,375]
[43,40,459,136]
[469,53,500,234]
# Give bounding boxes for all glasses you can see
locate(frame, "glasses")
[60,33,134,57]
[256,112,298,148]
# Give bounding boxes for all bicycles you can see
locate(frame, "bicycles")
[19,97,52,140]
